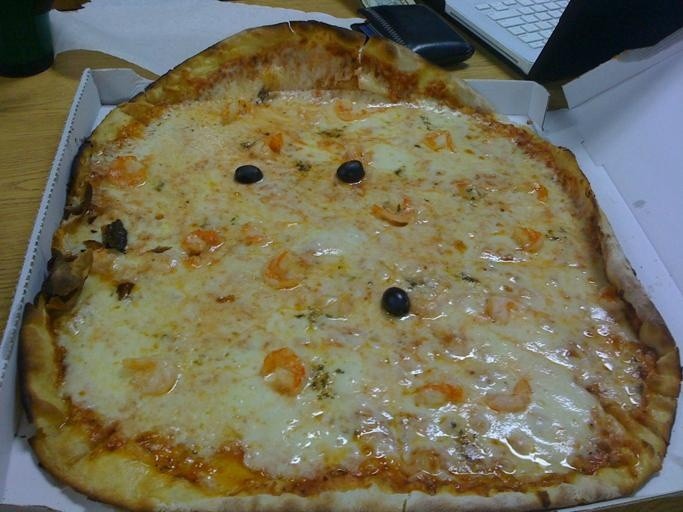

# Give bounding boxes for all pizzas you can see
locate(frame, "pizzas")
[19,23,683,512]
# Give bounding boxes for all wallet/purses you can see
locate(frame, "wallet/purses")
[351,4,475,68]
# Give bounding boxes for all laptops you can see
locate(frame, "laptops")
[440,0,682,84]
